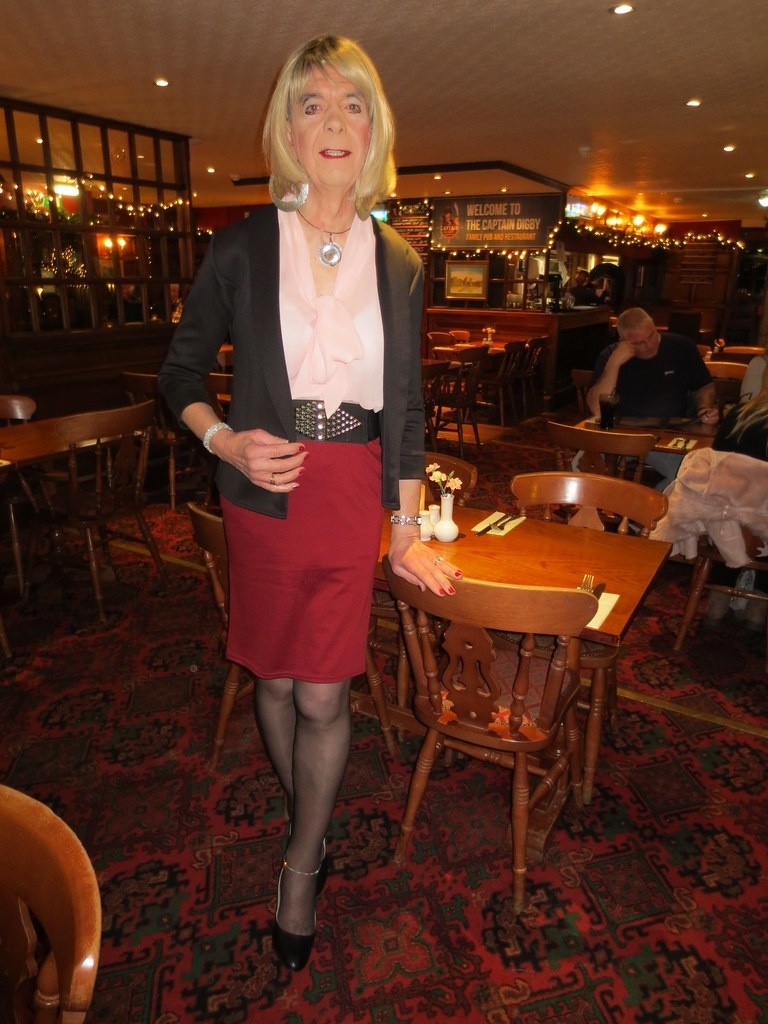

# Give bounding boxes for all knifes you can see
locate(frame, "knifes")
[475,512,512,536]
[591,583,606,600]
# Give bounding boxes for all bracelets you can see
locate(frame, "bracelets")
[390,515,421,526]
[203,423,233,454]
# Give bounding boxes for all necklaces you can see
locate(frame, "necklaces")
[297,210,351,267]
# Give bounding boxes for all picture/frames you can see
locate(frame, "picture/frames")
[444,259,490,301]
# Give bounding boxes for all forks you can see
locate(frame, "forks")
[489,515,521,533]
[581,573,594,593]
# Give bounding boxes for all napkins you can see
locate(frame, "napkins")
[469,512,527,536]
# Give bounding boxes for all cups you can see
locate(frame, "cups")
[599,391,620,430]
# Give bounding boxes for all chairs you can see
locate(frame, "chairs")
[0,783,101,1024]
[1,331,768,915]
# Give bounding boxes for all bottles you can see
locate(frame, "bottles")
[428,505,441,526]
[419,510,434,542]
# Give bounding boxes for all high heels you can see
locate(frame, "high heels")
[271,852,330,969]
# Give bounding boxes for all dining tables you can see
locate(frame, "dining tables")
[0,428,144,581]
[377,492,672,805]
[446,338,529,418]
[421,356,473,438]
[566,414,716,475]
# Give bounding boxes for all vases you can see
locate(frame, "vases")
[434,493,460,542]
[488,334,491,341]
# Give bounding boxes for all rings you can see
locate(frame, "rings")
[434,557,443,566]
[270,474,275,485]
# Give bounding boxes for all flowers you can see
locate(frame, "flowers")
[481,327,496,334]
[424,461,464,496]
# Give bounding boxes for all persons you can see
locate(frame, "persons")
[586,307,720,491]
[157,34,463,972]
[698,364,768,653]
[569,268,595,305]
